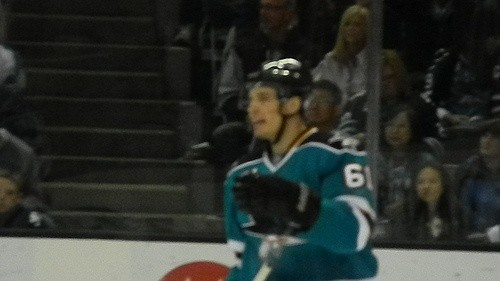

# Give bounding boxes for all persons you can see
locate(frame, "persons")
[308,4,380,105]
[423,9,500,139]
[212,0,316,181]
[365,104,444,222]
[452,127,500,244]
[326,49,441,156]
[0,44,57,228]
[387,162,471,245]
[302,79,343,150]
[222,57,378,281]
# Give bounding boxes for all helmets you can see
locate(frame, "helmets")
[248,57,313,91]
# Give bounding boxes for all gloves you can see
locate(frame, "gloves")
[233,175,300,218]
